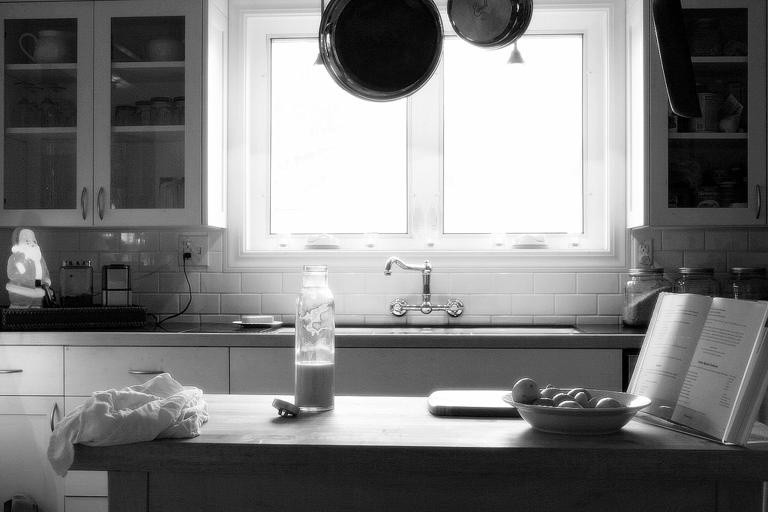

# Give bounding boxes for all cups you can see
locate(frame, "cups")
[157,177,175,208]
[177,176,185,207]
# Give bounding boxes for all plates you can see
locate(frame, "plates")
[232,320,283,327]
[502,388,652,438]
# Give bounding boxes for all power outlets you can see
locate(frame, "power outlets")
[177,234,210,267]
[636,236,654,266]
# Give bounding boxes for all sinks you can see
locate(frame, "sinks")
[426,325,587,336]
[265,324,425,332]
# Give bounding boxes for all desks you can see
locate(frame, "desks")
[70,393,768,512]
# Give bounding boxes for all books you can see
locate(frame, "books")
[624,291,768,447]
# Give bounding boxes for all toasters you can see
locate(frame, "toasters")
[101,264,132,307]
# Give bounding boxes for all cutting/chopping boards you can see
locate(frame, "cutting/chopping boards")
[428,389,518,418]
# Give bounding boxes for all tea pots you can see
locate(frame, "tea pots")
[19,29,75,64]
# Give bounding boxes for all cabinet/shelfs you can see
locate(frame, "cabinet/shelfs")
[0,0,228,232]
[626,0,765,228]
[0,345,231,512]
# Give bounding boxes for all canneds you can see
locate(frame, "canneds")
[624,266,667,326]
[676,266,720,295]
[727,266,767,299]
[690,93,718,132]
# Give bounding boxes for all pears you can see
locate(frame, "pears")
[512,378,629,409]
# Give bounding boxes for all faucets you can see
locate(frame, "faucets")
[383,256,431,302]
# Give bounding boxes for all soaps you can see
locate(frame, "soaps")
[242,313,274,324]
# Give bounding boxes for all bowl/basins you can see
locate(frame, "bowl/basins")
[447,0,533,51]
[318,0,446,102]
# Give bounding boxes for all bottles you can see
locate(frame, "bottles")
[115,96,185,126]
[60,260,94,305]
[294,265,335,412]
[622,266,767,329]
[143,37,185,61]
[669,164,748,208]
